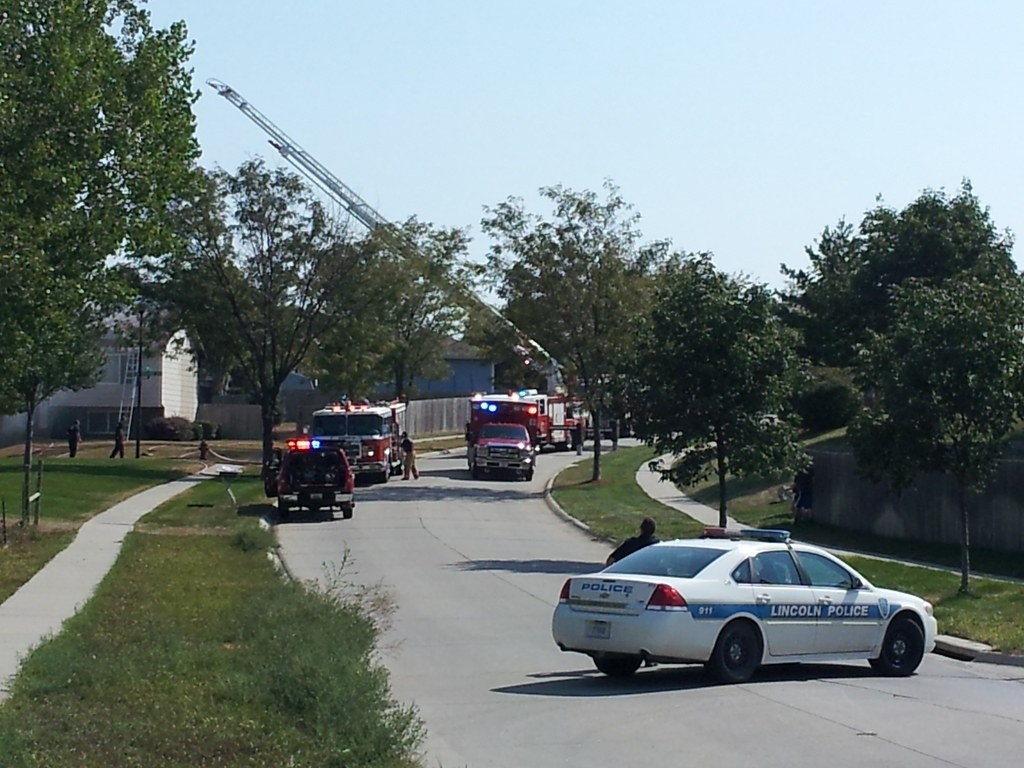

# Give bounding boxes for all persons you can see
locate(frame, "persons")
[396,431,419,480]
[66,420,82,457]
[110,422,124,458]
[574,423,583,455]
[606,517,660,566]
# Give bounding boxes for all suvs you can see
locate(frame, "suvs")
[468,422,541,480]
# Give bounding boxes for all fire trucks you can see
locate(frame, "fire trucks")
[301,394,409,487]
[202,76,636,455]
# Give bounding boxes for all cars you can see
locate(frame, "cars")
[262,436,357,523]
[542,515,939,685]
